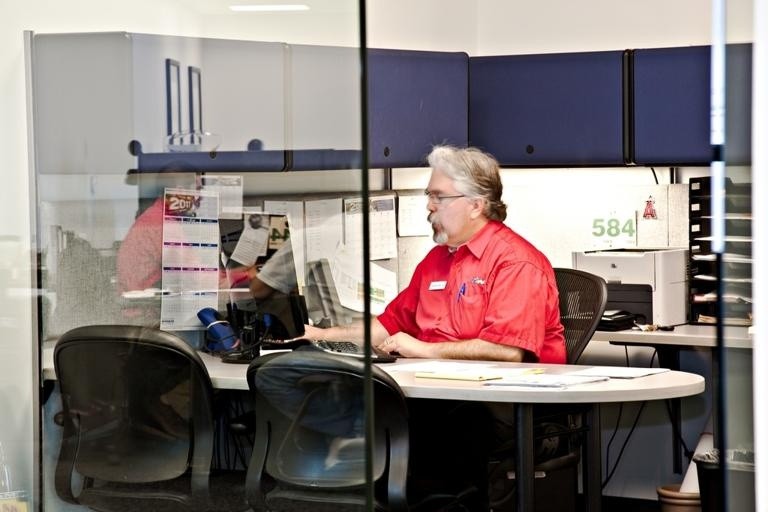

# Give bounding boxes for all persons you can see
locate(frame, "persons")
[262,142,569,366]
[244,194,367,472]
[115,162,264,444]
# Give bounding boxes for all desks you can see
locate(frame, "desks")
[51,335,707,509]
[590,318,753,512]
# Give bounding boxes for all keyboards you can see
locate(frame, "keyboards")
[311,338,396,363]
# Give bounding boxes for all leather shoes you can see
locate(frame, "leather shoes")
[141,394,188,437]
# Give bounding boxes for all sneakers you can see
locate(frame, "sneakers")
[323,433,367,471]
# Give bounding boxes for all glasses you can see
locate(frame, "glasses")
[424,190,462,205]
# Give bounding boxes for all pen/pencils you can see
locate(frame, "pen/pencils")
[458,283,465,299]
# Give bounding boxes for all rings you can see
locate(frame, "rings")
[384,337,391,344]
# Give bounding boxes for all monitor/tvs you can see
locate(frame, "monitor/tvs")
[217,211,306,364]
[304,257,347,329]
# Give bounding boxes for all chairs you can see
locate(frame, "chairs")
[55,239,157,327]
[449,268,607,512]
[54,325,257,511]
[243,351,413,511]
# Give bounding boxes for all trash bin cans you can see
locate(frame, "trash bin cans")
[692,449,754,512]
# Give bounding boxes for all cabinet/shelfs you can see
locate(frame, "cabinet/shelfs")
[630,44,753,167]
[469,49,631,169]
[288,46,468,166]
[35,33,286,172]
